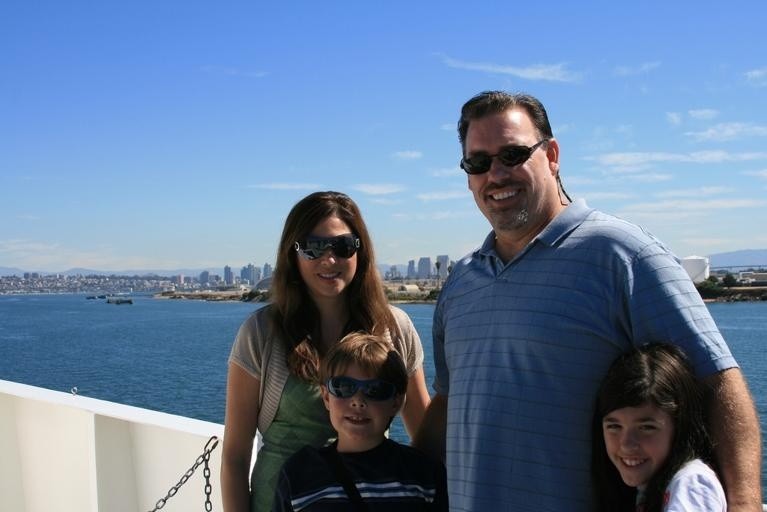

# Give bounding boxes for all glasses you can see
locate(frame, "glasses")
[293,231,363,263]
[324,374,397,405]
[459,137,551,175]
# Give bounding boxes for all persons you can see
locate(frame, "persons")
[219,191,434,510]
[269,330,441,512]
[411,89,764,512]
[590,337,731,512]
